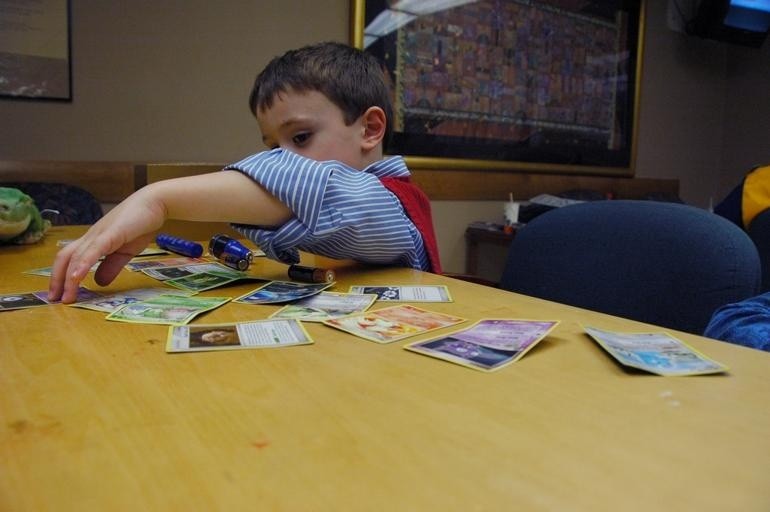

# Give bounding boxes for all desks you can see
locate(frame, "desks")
[465,219,512,281]
[0,223,767,512]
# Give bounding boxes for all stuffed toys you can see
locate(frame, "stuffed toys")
[0,187,54,246]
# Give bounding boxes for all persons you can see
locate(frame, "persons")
[46,39,441,306]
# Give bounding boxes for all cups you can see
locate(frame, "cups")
[502,200,520,234]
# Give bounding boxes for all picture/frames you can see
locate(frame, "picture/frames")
[349,0,646,175]
[0,0,74,103]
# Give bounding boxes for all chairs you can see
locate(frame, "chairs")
[502,197,762,342]
